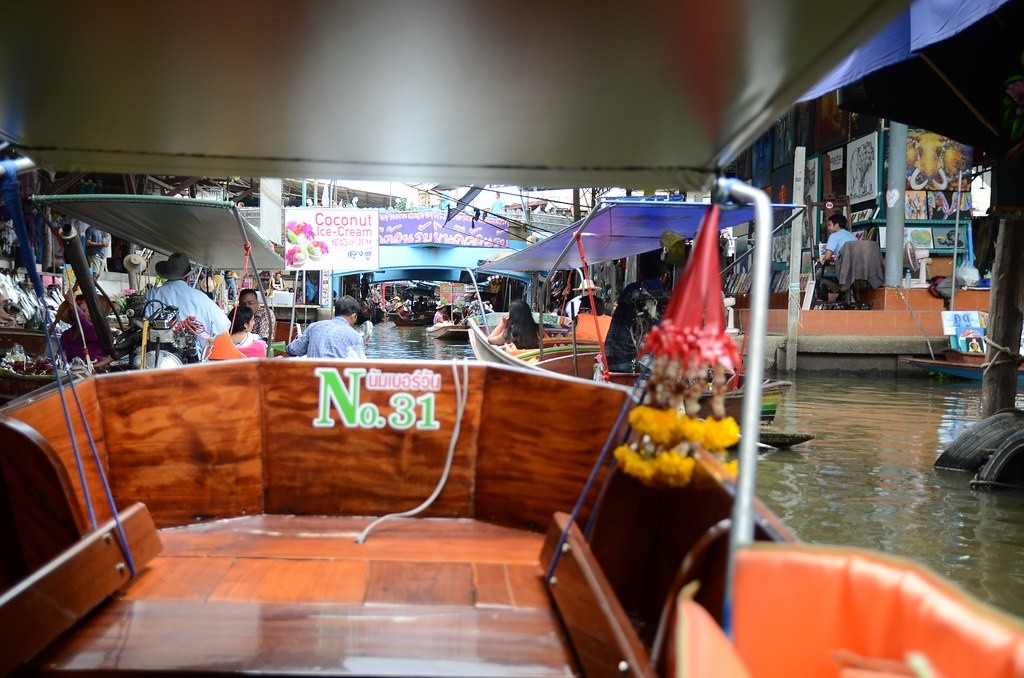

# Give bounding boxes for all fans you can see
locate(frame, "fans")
[905,241,931,288]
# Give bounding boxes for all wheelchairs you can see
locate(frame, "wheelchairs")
[814,241,881,309]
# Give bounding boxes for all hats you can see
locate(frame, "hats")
[201,276,216,292]
[436,299,452,309]
[154,251,198,280]
[58,293,112,325]
[227,271,240,280]
[123,254,147,274]
[572,279,602,291]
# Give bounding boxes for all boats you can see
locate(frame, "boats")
[387,285,437,326]
[0,193,300,406]
[426,325,470,339]
[468,309,817,449]
[900,348,1024,387]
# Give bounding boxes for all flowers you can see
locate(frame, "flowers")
[612,406,740,493]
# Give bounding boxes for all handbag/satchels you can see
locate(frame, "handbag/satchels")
[953,259,981,286]
[928,275,958,299]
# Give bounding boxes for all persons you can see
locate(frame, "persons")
[289,288,294,293]
[929,194,946,215]
[436,190,450,210]
[433,305,451,324]
[205,305,266,361]
[551,307,568,317]
[568,294,612,342]
[532,302,540,312]
[906,194,927,219]
[938,230,963,247]
[55,288,113,368]
[239,202,245,207]
[59,222,111,293]
[454,292,495,325]
[601,253,671,372]
[268,273,286,291]
[229,288,234,300]
[814,214,860,309]
[142,251,232,361]
[490,193,504,216]
[572,279,605,316]
[228,289,276,343]
[286,295,365,358]
[487,299,539,351]
[367,291,427,319]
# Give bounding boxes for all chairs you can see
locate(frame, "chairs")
[819,241,882,310]
